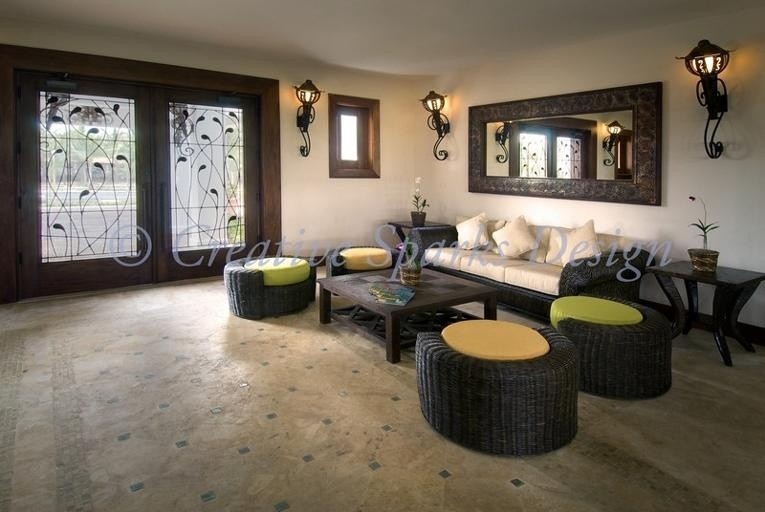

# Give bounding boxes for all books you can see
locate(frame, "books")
[374,286,416,307]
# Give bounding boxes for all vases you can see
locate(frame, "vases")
[688,249,719,272]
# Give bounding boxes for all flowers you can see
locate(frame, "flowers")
[687,195,720,248]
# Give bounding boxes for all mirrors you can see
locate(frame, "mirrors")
[467,81,662,205]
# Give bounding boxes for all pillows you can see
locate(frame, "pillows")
[492,215,538,258]
[554,220,601,269]
[456,213,489,249]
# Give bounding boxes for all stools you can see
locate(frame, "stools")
[411,319,580,455]
[545,292,673,398]
[223,256,316,320]
[326,246,406,310]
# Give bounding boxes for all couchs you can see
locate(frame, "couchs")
[408,224,655,326]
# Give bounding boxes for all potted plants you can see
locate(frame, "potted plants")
[411,194,429,226]
[400,231,421,286]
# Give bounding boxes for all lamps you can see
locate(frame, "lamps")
[495,120,515,163]
[602,120,624,165]
[674,38,729,158]
[420,89,451,161]
[292,79,325,157]
